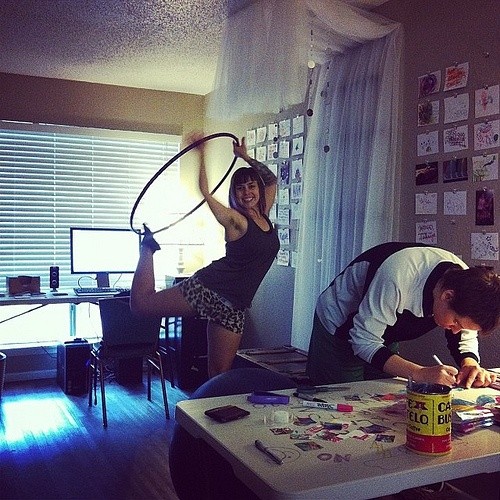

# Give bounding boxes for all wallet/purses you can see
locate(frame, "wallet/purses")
[204,405,250,422]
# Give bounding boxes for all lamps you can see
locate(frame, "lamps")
[155,212,205,275]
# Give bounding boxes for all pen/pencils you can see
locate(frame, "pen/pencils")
[433,354,457,383]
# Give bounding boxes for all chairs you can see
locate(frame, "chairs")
[88,299,169,428]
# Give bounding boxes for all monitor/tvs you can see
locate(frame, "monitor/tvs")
[70,227,141,274]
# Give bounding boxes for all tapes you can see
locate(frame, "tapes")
[273,411,288,423]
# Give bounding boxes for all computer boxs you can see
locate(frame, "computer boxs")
[57,344,91,395]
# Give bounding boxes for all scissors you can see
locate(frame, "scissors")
[296,383,351,394]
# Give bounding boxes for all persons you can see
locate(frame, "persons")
[306,242,500,389]
[478,193,493,225]
[129,127,280,379]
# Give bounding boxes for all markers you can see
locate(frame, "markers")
[255,440,284,465]
[293,392,328,403]
[302,400,353,412]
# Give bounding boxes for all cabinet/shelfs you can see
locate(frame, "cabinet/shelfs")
[159,275,209,388]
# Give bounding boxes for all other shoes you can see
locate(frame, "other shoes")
[141,223,161,250]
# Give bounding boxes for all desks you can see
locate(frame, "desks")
[175,368,500,499]
[0,286,176,388]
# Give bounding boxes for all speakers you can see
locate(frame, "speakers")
[50,266,59,292]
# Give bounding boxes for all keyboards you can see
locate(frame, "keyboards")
[73,287,129,296]
